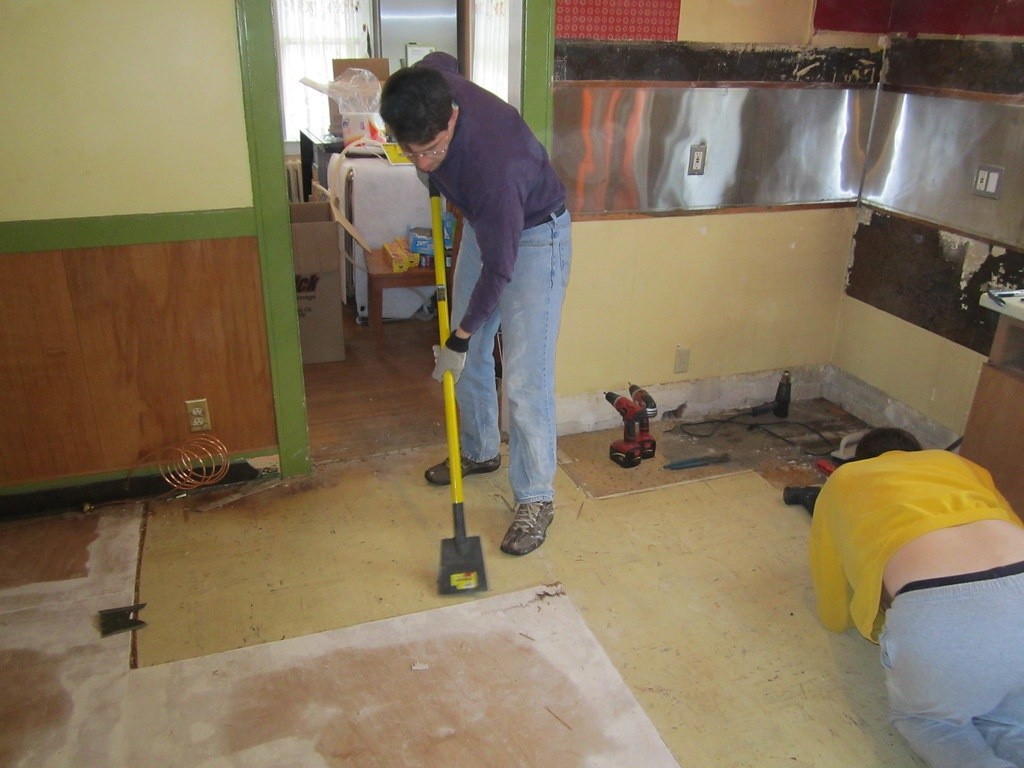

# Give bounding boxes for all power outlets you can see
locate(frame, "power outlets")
[676,347,689,372]
[185,398,211,432]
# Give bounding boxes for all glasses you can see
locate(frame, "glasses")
[398,124,451,158]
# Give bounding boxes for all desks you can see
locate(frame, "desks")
[299,129,344,202]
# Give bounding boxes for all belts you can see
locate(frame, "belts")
[533,204,566,226]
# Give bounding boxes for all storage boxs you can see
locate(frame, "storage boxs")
[290,201,372,365]
[298,56,389,137]
[382,211,456,272]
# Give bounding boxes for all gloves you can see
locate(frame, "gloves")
[431,329,472,384]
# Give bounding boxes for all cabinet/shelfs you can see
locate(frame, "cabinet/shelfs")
[955,292,1024,521]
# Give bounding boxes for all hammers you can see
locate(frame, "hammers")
[987,288,1024,307]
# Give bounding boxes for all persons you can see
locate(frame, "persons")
[808,428,1024,768]
[379,50,572,555]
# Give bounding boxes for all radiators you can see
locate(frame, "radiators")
[286,159,304,202]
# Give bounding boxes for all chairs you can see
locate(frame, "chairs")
[363,199,462,346]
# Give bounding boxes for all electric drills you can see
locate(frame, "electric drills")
[601,381,658,468]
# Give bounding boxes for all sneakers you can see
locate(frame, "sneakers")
[501,500,554,556]
[425,452,501,485]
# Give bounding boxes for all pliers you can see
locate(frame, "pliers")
[663,453,728,469]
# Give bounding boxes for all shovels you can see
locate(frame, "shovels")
[425,180,490,594]
